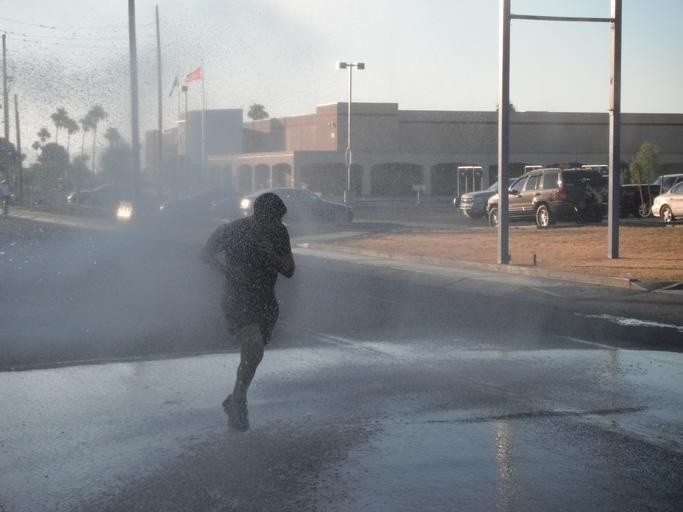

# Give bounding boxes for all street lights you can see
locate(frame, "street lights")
[181,85,190,156]
[338,63,365,188]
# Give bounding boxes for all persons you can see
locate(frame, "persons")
[0,176,12,216]
[200,192,294,430]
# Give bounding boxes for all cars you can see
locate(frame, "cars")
[454,164,682,227]
[61,173,353,235]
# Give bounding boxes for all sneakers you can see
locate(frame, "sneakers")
[222,395,250,432]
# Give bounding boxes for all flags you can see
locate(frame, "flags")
[183,67,200,82]
[167,71,177,97]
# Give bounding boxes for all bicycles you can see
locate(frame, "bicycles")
[1,196,7,218]
